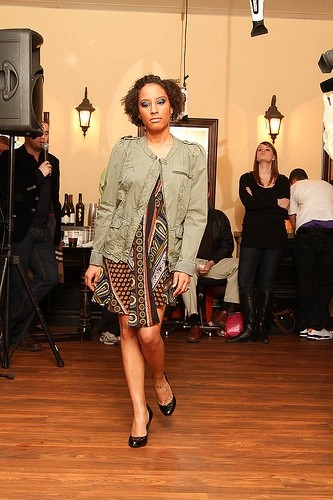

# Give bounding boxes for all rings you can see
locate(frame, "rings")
[188,287,190,289]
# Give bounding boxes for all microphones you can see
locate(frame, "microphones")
[42,143,49,161]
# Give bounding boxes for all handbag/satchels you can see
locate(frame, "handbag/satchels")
[272,307,299,335]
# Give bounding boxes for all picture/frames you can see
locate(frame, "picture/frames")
[138,118,219,208]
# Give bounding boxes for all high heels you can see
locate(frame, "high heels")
[152,373,176,416]
[129,404,152,448]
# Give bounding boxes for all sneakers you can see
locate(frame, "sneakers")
[99,332,118,345]
[307,329,332,339]
[300,328,307,337]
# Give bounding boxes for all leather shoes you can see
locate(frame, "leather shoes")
[188,325,202,343]
[11,336,41,351]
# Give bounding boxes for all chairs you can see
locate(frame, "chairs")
[164,277,226,336]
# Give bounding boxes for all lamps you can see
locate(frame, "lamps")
[75,86,97,137]
[264,94,285,140]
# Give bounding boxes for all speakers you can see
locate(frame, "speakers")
[0,28,44,140]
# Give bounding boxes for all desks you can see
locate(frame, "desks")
[31,247,91,340]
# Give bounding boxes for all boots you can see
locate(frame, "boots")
[225,290,258,342]
[254,291,270,343]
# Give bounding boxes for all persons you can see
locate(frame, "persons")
[226,142,290,344]
[179,181,240,343]
[288,168,333,340]
[84,75,208,449]
[98,309,120,345]
[0,123,62,351]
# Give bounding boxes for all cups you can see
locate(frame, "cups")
[67,231,80,247]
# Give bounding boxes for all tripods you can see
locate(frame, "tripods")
[0,135,66,368]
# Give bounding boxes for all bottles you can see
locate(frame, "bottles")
[76,193,84,226]
[61,194,70,225]
[88,203,97,225]
[68,195,75,226]
